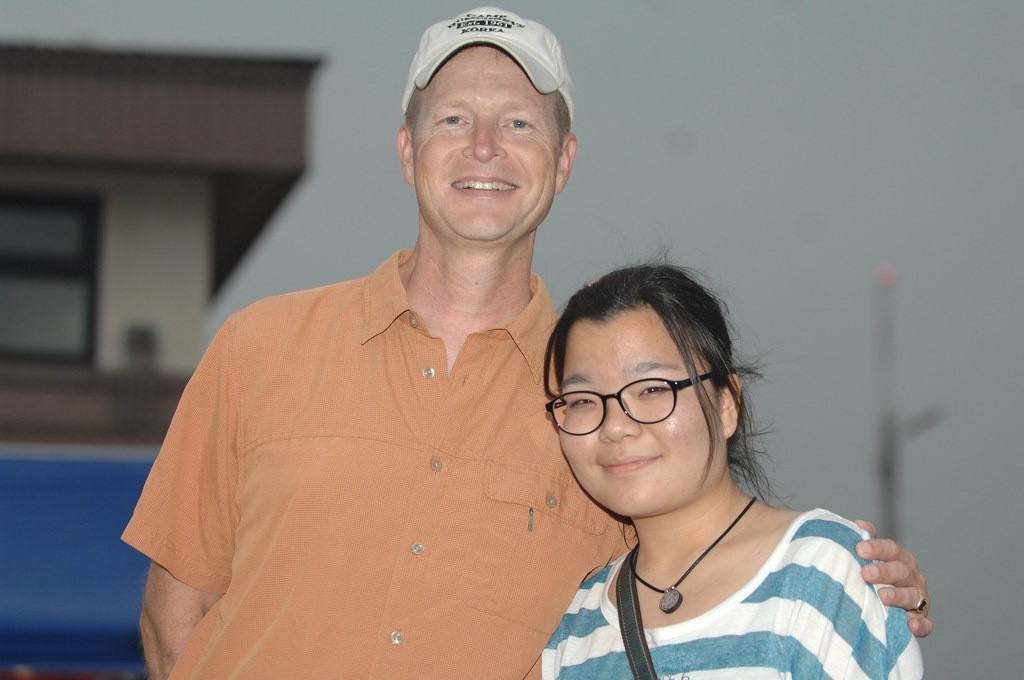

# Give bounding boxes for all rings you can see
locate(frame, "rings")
[909,588,928,614]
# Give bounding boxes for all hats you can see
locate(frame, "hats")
[399,5,574,126]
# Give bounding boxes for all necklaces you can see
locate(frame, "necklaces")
[627,496,756,614]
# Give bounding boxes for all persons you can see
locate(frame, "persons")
[118,5,641,679]
[540,261,922,680]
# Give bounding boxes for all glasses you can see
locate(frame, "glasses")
[545,371,729,436]
